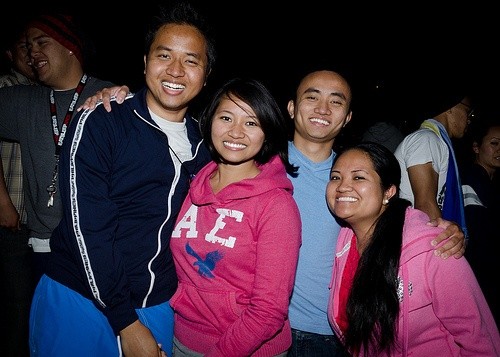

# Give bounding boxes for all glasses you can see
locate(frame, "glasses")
[458,101,475,117]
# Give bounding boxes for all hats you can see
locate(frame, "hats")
[425,75,466,117]
[29,10,83,58]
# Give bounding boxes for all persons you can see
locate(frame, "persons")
[29,8,212,357]
[76,76,302,357]
[0,11,118,270]
[326,142,500,356]
[286,66,465,357]
[464,118,500,332]
[0,15,43,357]
[392,81,474,238]
[361,120,404,149]
